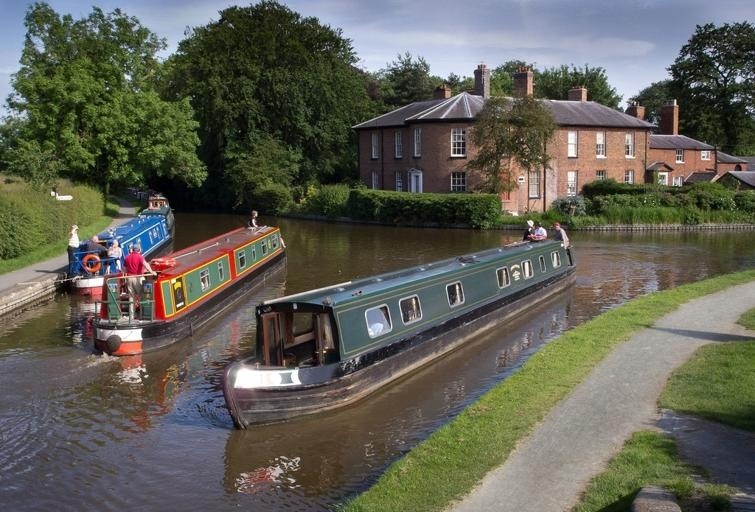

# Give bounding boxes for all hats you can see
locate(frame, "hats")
[527,220,534,228]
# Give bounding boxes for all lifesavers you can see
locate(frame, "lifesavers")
[82,255,101,272]
[150,257,177,266]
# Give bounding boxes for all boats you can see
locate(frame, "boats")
[92,227,287,356]
[64,217,174,295]
[129,183,174,231]
[222,238,578,431]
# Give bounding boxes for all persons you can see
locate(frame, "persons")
[122,245,157,319]
[533,221,548,238]
[248,210,258,227]
[104,239,122,274]
[65,225,79,276]
[79,235,107,277]
[553,221,570,250]
